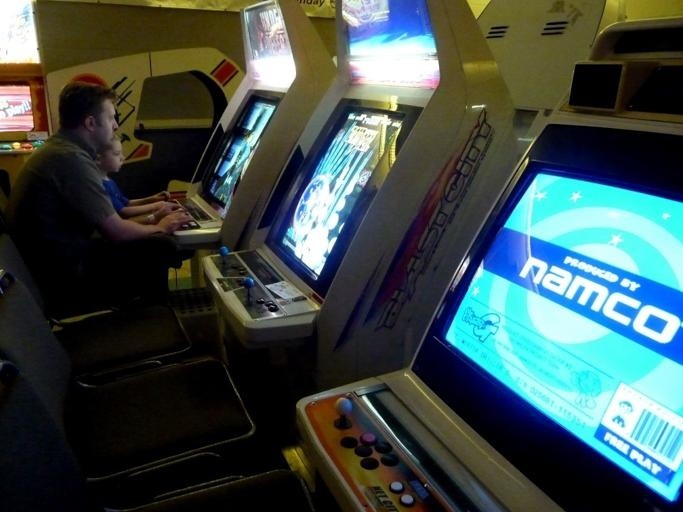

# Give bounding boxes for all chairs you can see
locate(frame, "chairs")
[2,184,148,328]
[4,233,195,382]
[132,468,319,508]
[3,271,258,482]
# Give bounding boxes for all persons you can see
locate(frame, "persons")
[2,81,195,321]
[95,135,182,217]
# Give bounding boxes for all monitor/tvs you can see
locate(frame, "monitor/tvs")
[431,161,682,503]
[0,80,35,132]
[275,107,405,279]
[203,99,276,208]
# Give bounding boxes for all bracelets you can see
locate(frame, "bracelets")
[148,214,155,224]
